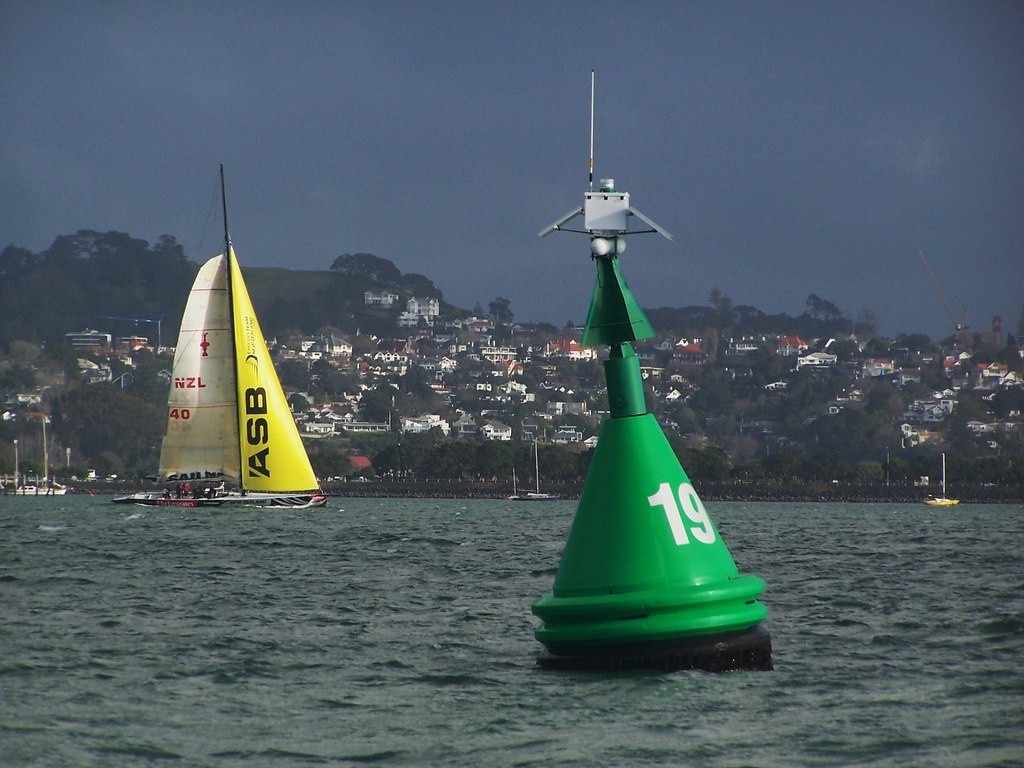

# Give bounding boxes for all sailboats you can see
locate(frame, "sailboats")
[15,418,67,497]
[924,453,961,506]
[507,468,520,500]
[509,437,560,501]
[110,171,328,509]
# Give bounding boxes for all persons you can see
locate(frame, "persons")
[161,479,229,498]
